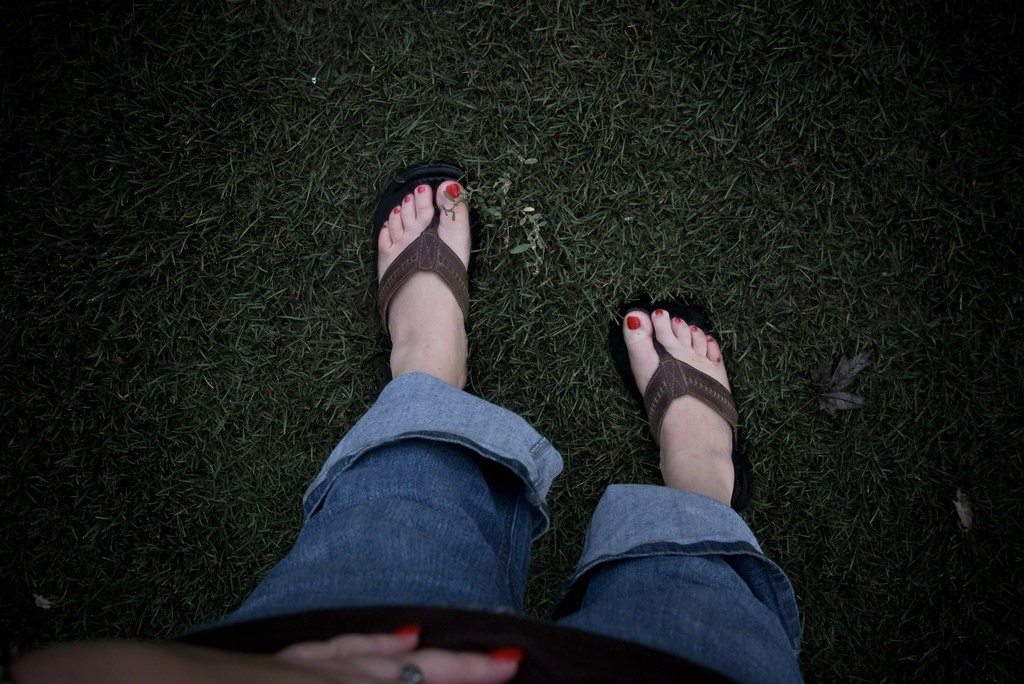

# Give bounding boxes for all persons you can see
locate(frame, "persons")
[0,162,801,684]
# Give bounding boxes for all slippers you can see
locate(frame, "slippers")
[368,160,475,381]
[608,294,754,511]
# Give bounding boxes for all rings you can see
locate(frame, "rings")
[401,663,424,684]
